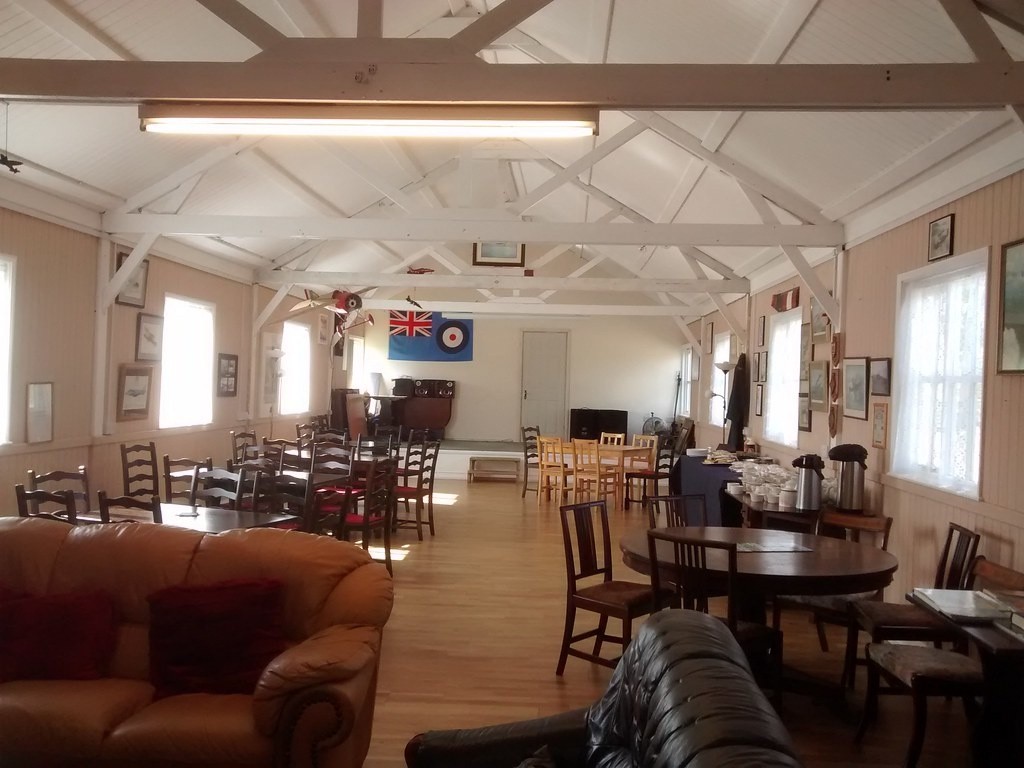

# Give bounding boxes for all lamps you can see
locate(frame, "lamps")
[137,100,600,140]
[704,361,737,444]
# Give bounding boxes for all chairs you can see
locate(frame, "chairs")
[14,414,1024,768]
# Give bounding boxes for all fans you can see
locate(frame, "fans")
[642,417,668,448]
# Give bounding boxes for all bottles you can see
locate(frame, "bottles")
[706,447,713,460]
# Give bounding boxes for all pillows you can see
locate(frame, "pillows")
[143,576,289,703]
[0,582,118,684]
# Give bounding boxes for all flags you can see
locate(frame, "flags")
[389,310,473,361]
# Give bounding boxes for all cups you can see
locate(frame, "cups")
[727,466,838,509]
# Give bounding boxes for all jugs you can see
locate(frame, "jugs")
[827,443,869,512]
[790,453,825,510]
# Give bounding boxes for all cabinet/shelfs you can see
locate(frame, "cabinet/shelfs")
[331,388,360,429]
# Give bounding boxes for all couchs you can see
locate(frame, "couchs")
[0,515,394,768]
[403,609,806,768]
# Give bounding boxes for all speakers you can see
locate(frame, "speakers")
[414,380,455,398]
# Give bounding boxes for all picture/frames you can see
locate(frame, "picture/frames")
[24,382,54,445]
[871,403,888,450]
[216,353,238,397]
[705,322,714,355]
[798,392,813,433]
[927,213,955,262]
[674,427,689,455]
[114,252,164,423]
[755,384,764,417]
[691,339,702,382]
[317,314,330,346]
[471,243,526,268]
[842,357,869,421]
[807,360,830,414]
[761,351,768,382]
[752,352,760,383]
[799,322,815,381]
[996,237,1024,377]
[758,316,765,347]
[809,290,833,344]
[869,357,892,397]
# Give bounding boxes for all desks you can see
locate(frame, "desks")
[542,441,655,514]
[163,466,352,542]
[669,452,876,540]
[569,409,627,445]
[364,394,407,437]
[61,502,305,534]
[259,449,405,540]
[619,527,900,709]
[904,591,1024,768]
